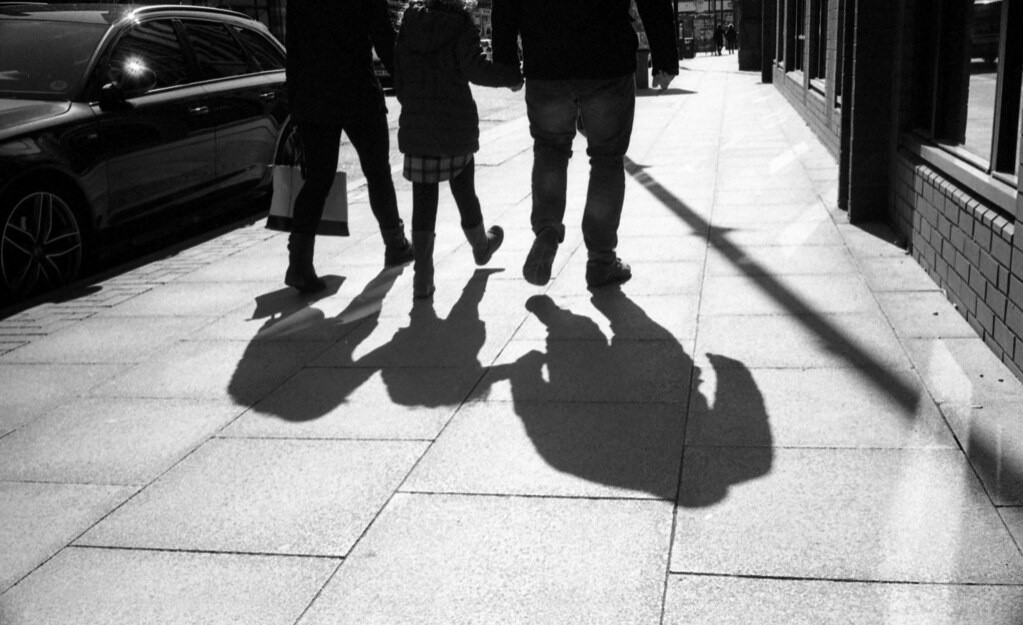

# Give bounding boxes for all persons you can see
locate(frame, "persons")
[281,1,415,293]
[491,0,680,288]
[726,24,737,54]
[396,0,524,297]
[714,24,726,55]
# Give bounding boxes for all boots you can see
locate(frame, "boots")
[462,215,503,264]
[377,219,415,266]
[412,231,436,298]
[283,234,327,292]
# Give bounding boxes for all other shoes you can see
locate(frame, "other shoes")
[586,251,633,286]
[523,227,559,287]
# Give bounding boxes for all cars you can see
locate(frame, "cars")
[0,1,292,307]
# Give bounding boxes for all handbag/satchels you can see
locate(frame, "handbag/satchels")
[261,114,350,237]
